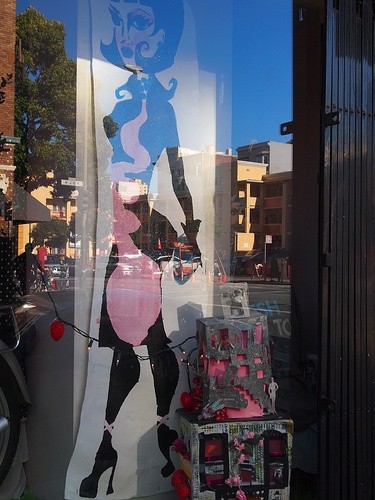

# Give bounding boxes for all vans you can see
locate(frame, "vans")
[93,256,138,277]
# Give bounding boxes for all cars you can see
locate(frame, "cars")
[147,255,188,273]
[181,256,218,273]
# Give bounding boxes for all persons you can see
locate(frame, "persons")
[11,243,47,296]
[36,241,48,272]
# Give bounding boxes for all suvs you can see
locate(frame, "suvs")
[43,254,74,276]
